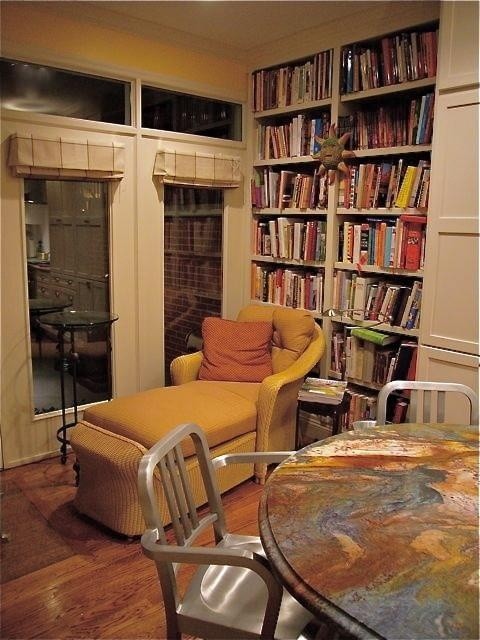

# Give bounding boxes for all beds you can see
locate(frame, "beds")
[70,304,325,545]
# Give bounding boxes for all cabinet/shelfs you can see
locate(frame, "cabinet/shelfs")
[166,188,221,384]
[253,20,439,429]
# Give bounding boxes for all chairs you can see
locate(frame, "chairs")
[137,422,315,639]
[376,380,479,426]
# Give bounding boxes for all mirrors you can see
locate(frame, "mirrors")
[162,184,228,389]
[22,177,115,420]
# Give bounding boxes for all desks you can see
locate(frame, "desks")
[258,423,479,640]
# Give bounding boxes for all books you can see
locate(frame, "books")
[330,270,422,328]
[252,165,328,209]
[331,326,418,396]
[338,159,430,208]
[297,377,408,450]
[337,93,434,150]
[255,114,331,160]
[338,214,425,271]
[255,217,326,262]
[341,30,437,95]
[251,49,332,112]
[253,264,323,314]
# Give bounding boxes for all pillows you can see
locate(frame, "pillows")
[197,316,274,383]
